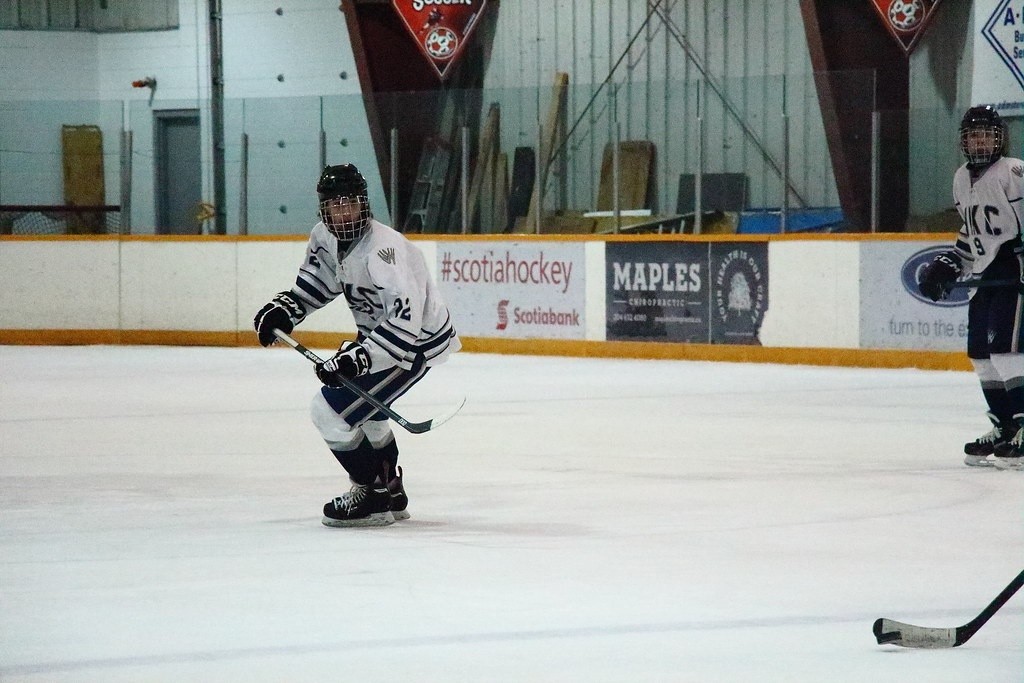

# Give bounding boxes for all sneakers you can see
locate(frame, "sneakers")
[386,465,411,520]
[994,413,1024,471]
[322,459,396,527]
[964,410,1016,467]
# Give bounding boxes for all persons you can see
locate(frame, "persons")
[918,104,1024,473]
[255,163,464,528]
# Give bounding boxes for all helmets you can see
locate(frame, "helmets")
[317,163,371,241]
[959,104,1004,167]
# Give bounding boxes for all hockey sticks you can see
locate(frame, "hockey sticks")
[872,570,1024,649]
[944,278,1024,295]
[272,327,468,435]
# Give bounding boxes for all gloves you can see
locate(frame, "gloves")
[1013,240,1024,296]
[316,341,371,387]
[254,291,307,347]
[918,250,962,303]
